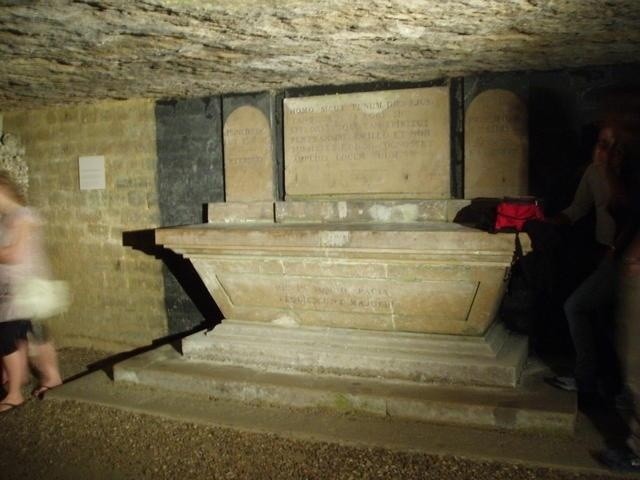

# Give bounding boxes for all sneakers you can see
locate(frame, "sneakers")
[542,371,581,394]
[593,443,640,471]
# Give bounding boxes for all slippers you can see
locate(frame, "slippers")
[0,395,28,413]
[35,377,65,399]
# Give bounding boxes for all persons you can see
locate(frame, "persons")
[529,114,640,394]
[1,169,66,414]
[594,237,640,475]
